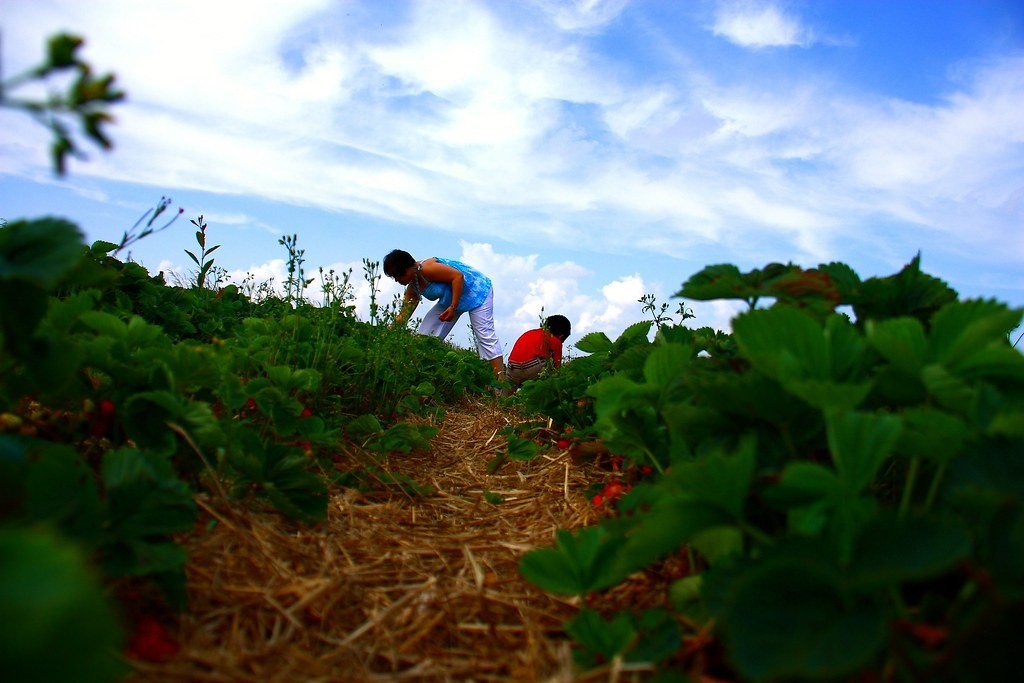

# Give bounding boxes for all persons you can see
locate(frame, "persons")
[383,249,505,384]
[506,314,571,388]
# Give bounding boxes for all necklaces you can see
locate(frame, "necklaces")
[410,270,423,304]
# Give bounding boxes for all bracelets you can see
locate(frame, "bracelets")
[450,304,458,311]
